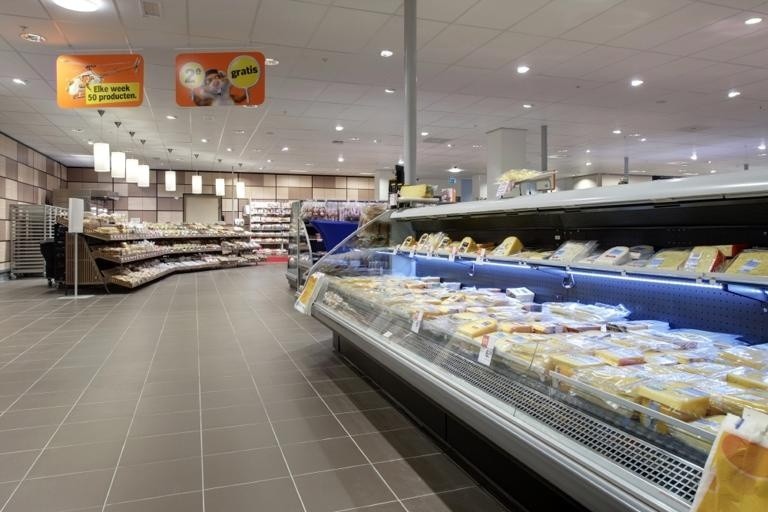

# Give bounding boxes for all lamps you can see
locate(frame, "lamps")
[522,103,534,109]
[164,114,177,120]
[88,138,94,144]
[516,64,530,74]
[744,17,762,26]
[111,151,125,178]
[93,142,110,172]
[727,87,740,98]
[202,138,208,145]
[334,124,344,132]
[632,79,644,85]
[448,166,463,173]
[126,158,138,183]
[236,180,245,199]
[383,88,396,94]
[137,164,148,187]
[164,170,177,192]
[379,49,394,59]
[215,179,225,197]
[191,174,202,194]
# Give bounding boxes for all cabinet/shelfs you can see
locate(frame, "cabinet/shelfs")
[63,198,261,294]
[293,167,768,512]
[288,201,388,289]
[244,198,291,262]
